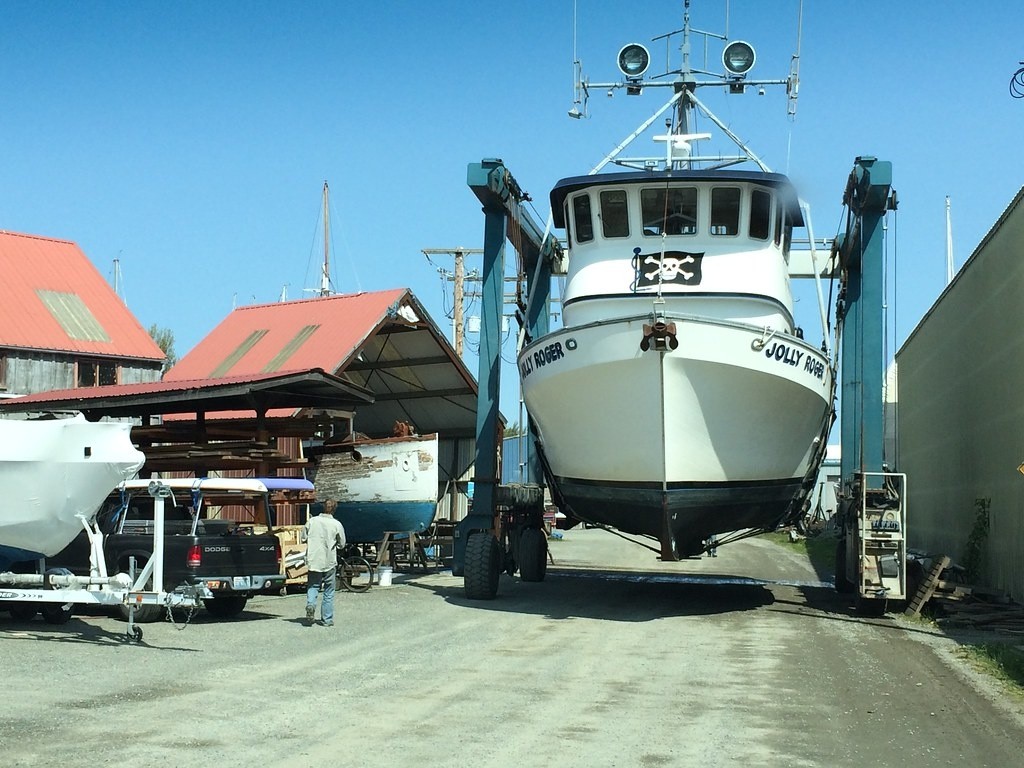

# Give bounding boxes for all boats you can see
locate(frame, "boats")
[512,1,839,563]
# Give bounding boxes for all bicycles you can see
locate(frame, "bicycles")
[334,548,374,593]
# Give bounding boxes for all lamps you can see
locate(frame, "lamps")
[721,41,756,95]
[617,42,650,95]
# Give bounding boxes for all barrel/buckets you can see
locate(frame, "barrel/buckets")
[377,566,393,586]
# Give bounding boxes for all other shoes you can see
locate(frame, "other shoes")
[306,605,315,625]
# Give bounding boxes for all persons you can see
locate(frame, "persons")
[300,497,346,627]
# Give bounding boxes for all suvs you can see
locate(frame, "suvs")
[0,489,288,624]
